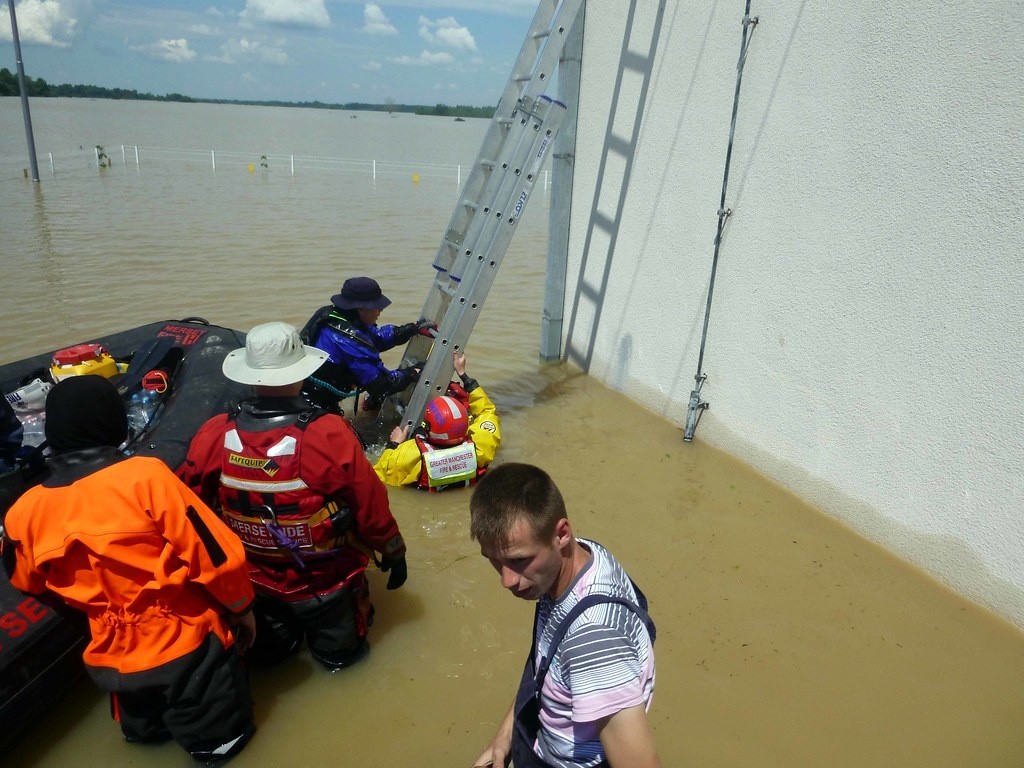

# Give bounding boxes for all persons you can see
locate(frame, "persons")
[373,348,501,494]
[175,326,407,689]
[468,462,660,768]
[0,374,254,767]
[299,276,438,417]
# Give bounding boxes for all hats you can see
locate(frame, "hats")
[45,375,128,455]
[222,322,330,386]
[331,277,392,311]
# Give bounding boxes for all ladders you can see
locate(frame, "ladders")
[374,0,587,442]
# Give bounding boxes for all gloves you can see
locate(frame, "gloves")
[398,360,425,387]
[380,551,407,590]
[415,318,438,338]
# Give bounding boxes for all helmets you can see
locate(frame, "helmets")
[421,396,469,446]
